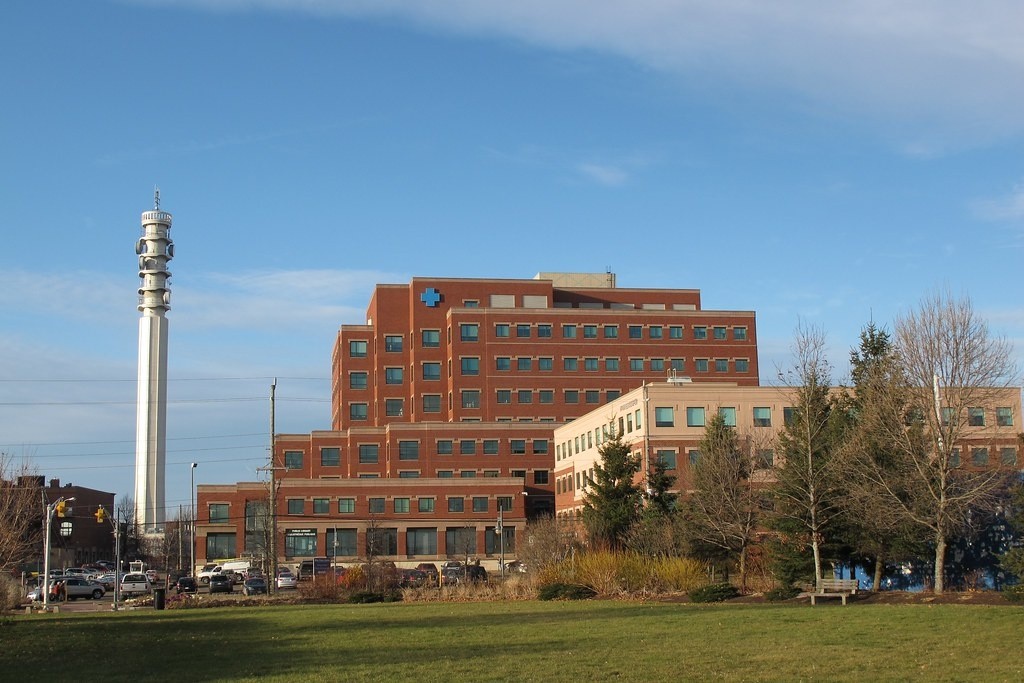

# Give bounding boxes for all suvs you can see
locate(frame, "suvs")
[167,569,188,591]
[295,561,314,582]
[241,566,264,580]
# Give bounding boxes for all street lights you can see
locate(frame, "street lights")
[495,504,505,581]
[42,497,77,611]
[190,462,198,578]
[99,503,121,611]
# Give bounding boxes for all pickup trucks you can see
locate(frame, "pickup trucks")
[197,564,246,584]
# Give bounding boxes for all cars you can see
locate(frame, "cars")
[275,572,298,588]
[240,576,268,596]
[312,556,529,590]
[177,577,199,596]
[120,572,153,600]
[25,562,123,603]
[146,569,160,585]
[207,574,232,595]
[217,568,238,586]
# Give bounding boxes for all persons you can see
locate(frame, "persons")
[497,556,502,571]
[475,557,480,565]
[53,579,67,602]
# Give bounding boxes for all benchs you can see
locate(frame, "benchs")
[801,579,859,605]
